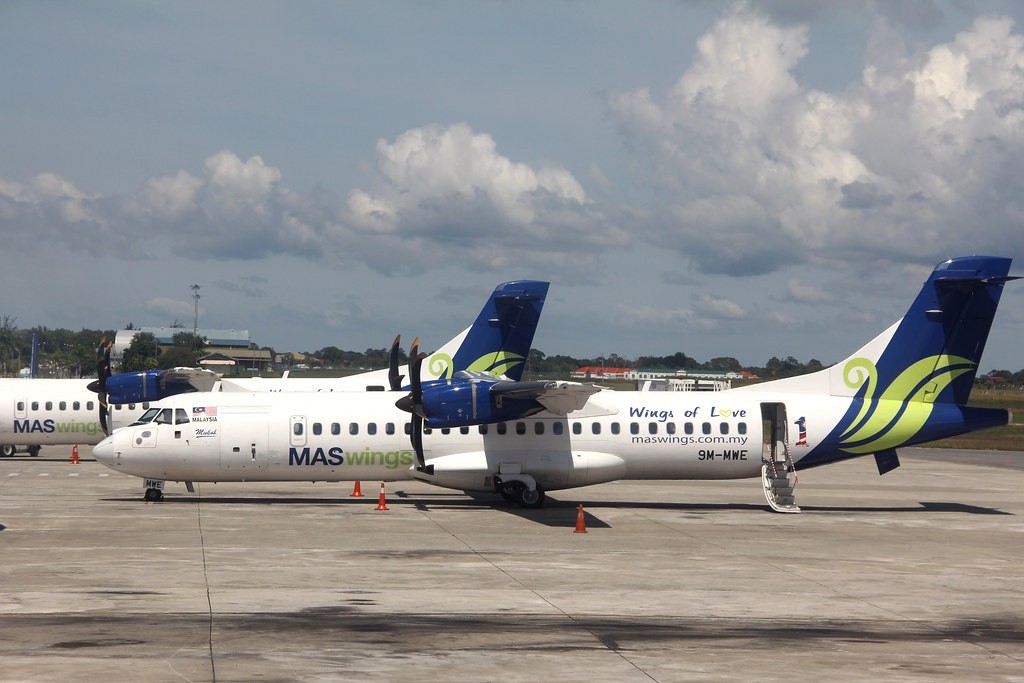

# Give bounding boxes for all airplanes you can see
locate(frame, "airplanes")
[0,280,552,457]
[92,255,1023,513]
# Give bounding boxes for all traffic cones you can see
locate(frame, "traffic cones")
[350,480,364,496]
[572,504,588,533]
[70,443,80,463]
[374,482,389,510]
